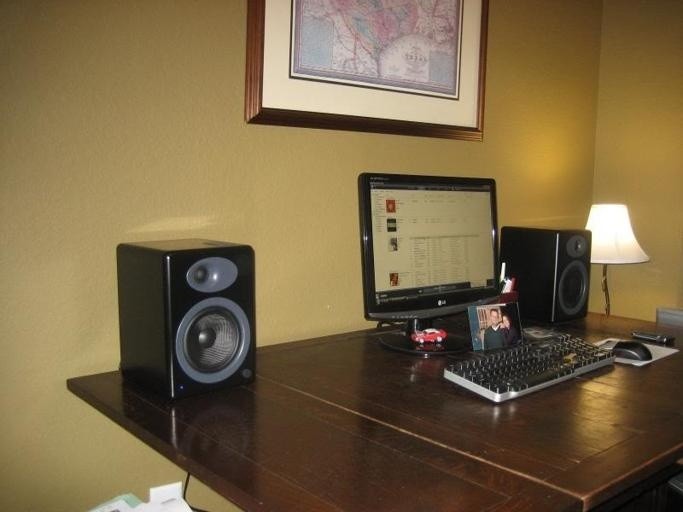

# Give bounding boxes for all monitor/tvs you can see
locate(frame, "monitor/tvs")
[358,171,498,358]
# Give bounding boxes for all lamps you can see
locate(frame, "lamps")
[585,202,651,317]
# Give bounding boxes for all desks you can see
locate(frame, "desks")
[66,310,683,510]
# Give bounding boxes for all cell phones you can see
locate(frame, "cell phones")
[631,329,677,345]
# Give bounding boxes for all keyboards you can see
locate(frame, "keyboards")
[444,331,616,403]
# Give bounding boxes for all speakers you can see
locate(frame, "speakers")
[116,237,258,400]
[500,225,591,322]
[131,401,256,497]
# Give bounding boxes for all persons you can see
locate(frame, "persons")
[483,308,518,350]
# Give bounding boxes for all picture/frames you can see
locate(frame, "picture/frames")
[244,0,489,141]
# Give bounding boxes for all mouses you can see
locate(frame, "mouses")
[612,341,652,361]
